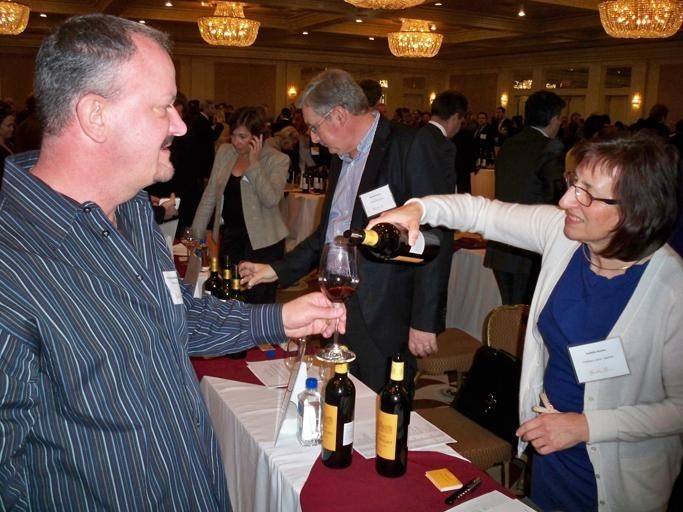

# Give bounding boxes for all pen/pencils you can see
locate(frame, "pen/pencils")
[444,477,482,505]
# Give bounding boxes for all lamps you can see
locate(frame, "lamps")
[0,0,32,37]
[302,31,309,35]
[287,84,298,98]
[138,20,146,24]
[343,0,425,11]
[435,3,442,6]
[197,1,261,47]
[518,10,526,16]
[384,18,444,59]
[500,92,508,108]
[164,1,173,7]
[596,0,683,39]
[429,90,436,104]
[630,92,642,110]
[368,37,374,41]
[355,19,363,23]
[39,13,47,18]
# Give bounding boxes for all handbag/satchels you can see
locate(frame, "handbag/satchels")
[451,344,522,455]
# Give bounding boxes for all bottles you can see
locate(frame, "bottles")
[219,254,233,300]
[322,346,355,468]
[197,239,210,267]
[203,256,222,299]
[344,222,440,268]
[375,354,411,477]
[226,265,247,360]
[296,377,322,448]
[302,164,309,192]
[312,165,320,192]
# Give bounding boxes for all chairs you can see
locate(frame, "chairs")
[414,326,482,392]
[413,303,531,490]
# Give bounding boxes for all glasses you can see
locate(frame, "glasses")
[565,171,620,207]
[307,107,336,133]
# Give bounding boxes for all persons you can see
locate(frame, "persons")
[170,91,332,189]
[366,130,683,512]
[190,107,290,305]
[148,192,179,225]
[461,107,523,167]
[357,79,383,107]
[1,92,42,152]
[556,103,683,171]
[389,106,429,127]
[237,67,455,409]
[0,108,16,189]
[419,89,468,333]
[0,13,347,512]
[482,91,567,307]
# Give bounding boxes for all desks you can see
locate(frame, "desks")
[162,237,535,512]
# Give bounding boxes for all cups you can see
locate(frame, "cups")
[315,243,360,366]
[182,226,198,266]
